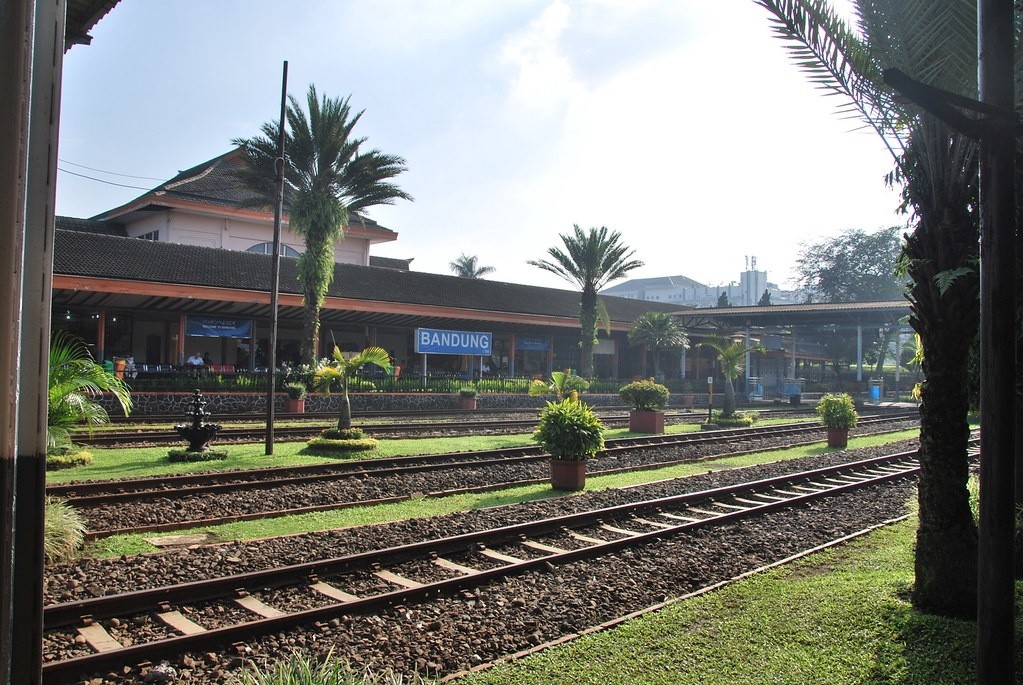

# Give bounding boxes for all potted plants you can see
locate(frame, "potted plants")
[286,383,307,413]
[459,387,477,410]
[530,396,606,490]
[815,392,860,448]
[618,376,670,434]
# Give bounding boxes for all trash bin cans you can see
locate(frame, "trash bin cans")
[868,379,884,403]
[102,360,114,375]
[384,366,395,384]
[114,361,126,380]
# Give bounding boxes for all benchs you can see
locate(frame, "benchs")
[137,364,184,372]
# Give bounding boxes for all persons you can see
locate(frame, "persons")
[188,352,213,378]
[483,363,490,376]
[123,352,138,378]
[399,361,408,372]
